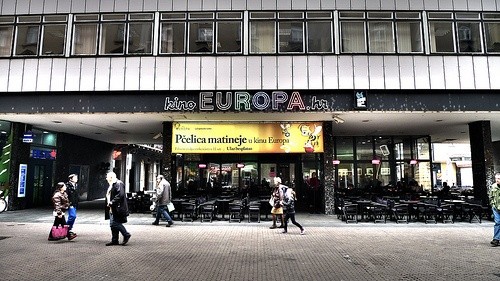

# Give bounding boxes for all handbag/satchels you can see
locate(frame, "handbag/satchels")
[269,195,279,206]
[271,207,285,215]
[52,225,68,239]
[167,202,175,213]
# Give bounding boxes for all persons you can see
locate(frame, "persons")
[338,181,348,197]
[48,181,77,241]
[151,175,173,227]
[387,173,421,199]
[488,171,500,246]
[64,173,80,241]
[269,177,285,229]
[348,183,358,197]
[376,180,386,198]
[104,171,131,246]
[280,181,305,234]
[439,181,451,201]
[308,171,320,206]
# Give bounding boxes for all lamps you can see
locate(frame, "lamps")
[153,131,163,140]
[333,116,344,125]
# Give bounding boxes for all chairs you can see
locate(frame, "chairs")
[335,189,492,224]
[171,196,262,223]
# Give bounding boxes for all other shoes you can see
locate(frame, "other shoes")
[278,225,284,228]
[270,224,276,228]
[152,221,159,224]
[165,222,173,227]
[282,230,287,233]
[105,241,120,246]
[300,229,306,235]
[122,233,131,245]
[68,233,76,240]
[491,239,500,246]
[48,236,59,241]
